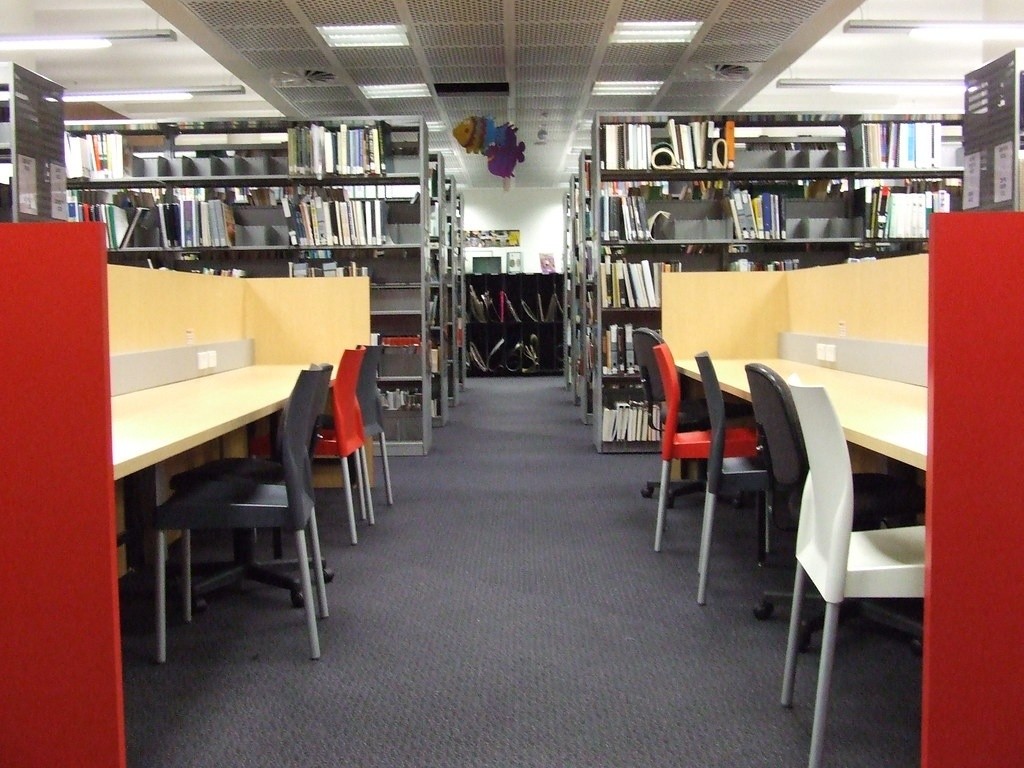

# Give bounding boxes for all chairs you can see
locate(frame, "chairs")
[628,327,926,768]
[144,342,384,666]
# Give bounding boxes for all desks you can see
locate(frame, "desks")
[674,357,928,470]
[112,361,335,484]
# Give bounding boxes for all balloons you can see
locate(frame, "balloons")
[452,117,525,181]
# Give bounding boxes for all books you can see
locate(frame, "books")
[66,121,438,410]
[566,119,1024,442]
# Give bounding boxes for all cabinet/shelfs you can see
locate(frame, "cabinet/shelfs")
[0,50,1021,455]
